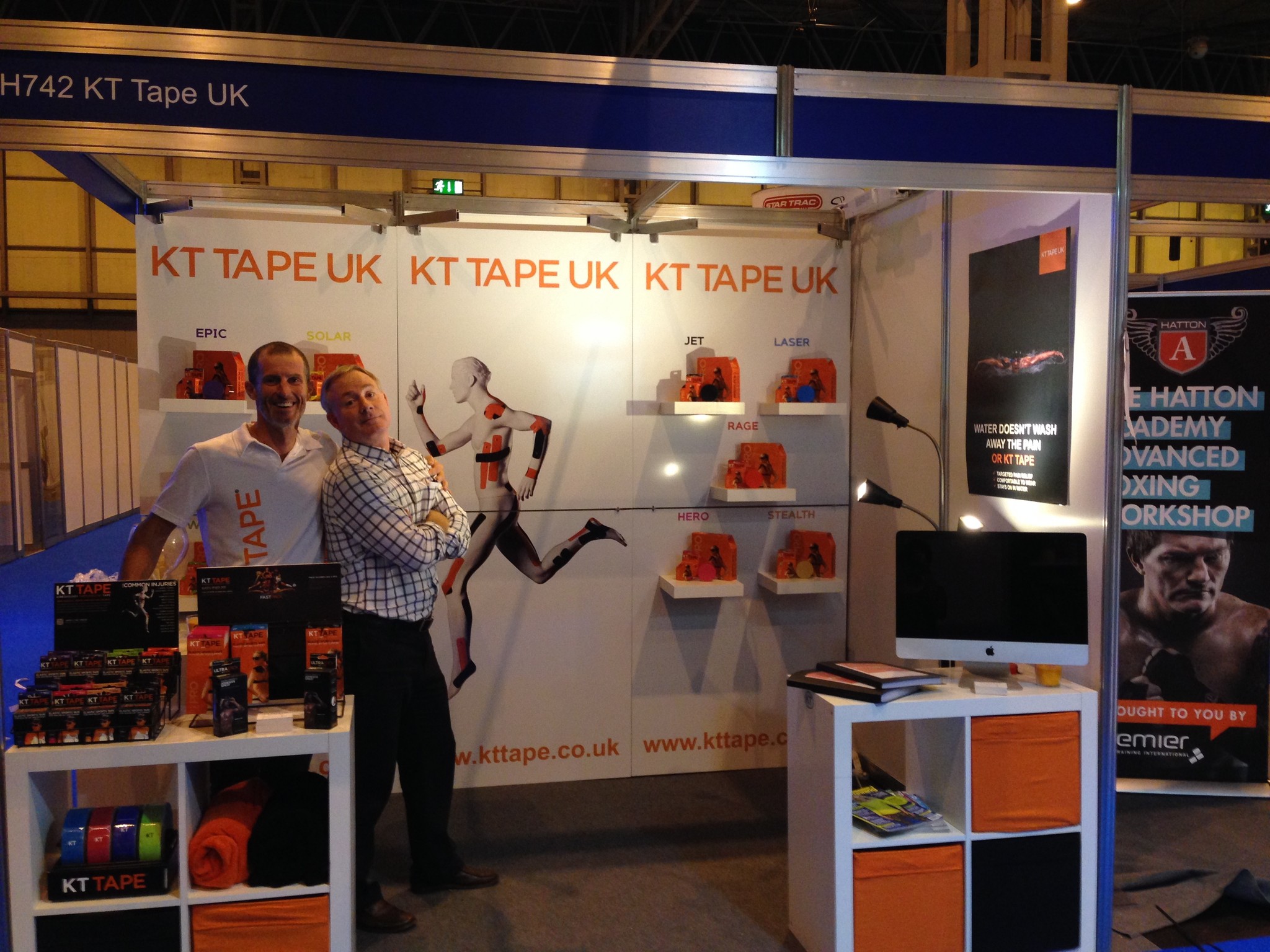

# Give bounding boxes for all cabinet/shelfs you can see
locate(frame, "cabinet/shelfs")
[656,402,846,598]
[5,694,357,952]
[159,398,325,613]
[787,673,1099,951]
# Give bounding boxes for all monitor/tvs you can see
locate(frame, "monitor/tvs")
[899,531,1089,670]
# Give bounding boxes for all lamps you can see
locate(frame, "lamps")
[856,397,981,533]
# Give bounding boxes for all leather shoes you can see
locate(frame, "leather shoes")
[409,865,498,892]
[355,901,416,933]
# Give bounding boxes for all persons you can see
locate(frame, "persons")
[785,562,796,579]
[687,385,697,401]
[886,796,934,819]
[220,696,244,736]
[711,367,731,402]
[733,471,742,489]
[783,386,792,403]
[203,676,212,714]
[320,366,499,936]
[185,380,194,399]
[854,793,925,826]
[115,340,446,812]
[710,545,727,580]
[212,361,233,399]
[809,543,829,577]
[1118,527,1270,735]
[809,369,823,403]
[758,453,778,488]
[24,714,149,744]
[683,564,693,581]
[248,650,269,704]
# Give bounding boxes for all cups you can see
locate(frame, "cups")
[1036,664,1061,686]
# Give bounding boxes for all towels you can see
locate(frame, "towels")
[187,779,270,889]
[247,773,329,886]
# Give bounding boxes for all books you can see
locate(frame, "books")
[852,786,951,833]
[786,661,948,703]
